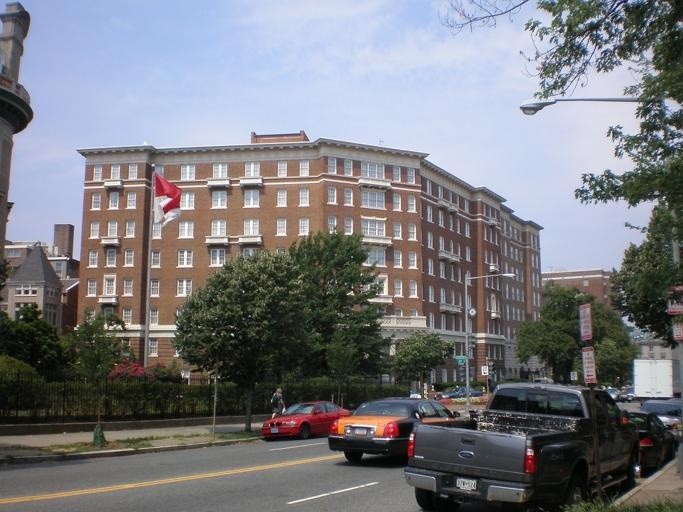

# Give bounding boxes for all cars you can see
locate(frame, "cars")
[209,370,223,383]
[641,399,683,443]
[327,394,478,463]
[262,400,350,440]
[620,408,679,479]
[604,386,638,402]
[434,385,484,400]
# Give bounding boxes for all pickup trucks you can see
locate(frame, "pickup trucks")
[402,382,644,511]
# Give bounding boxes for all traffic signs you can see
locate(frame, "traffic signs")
[666,285,683,314]
[456,355,466,366]
[579,302,594,341]
[672,319,683,341]
[582,346,598,383]
[481,366,489,375]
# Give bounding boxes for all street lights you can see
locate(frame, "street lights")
[518,97,681,284]
[463,273,519,398]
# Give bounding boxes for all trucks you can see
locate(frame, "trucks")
[632,358,682,405]
[532,376,553,385]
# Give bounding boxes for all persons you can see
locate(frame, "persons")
[271,388,286,419]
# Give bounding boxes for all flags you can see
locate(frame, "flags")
[151,171,181,227]
